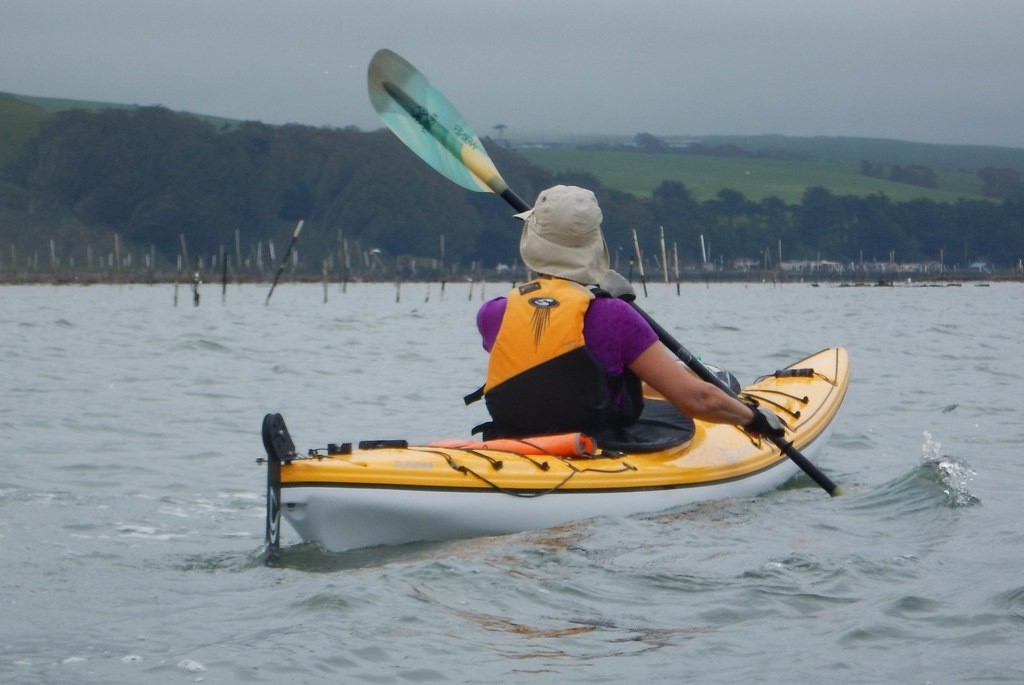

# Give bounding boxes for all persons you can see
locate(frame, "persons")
[477,186,786,441]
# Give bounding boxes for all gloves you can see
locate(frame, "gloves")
[744,404,786,437]
[598,269,637,301]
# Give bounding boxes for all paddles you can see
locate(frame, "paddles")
[366,48,850,500]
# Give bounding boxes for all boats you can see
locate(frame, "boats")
[259,345,849,563]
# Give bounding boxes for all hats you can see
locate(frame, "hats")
[512,185,610,285]
